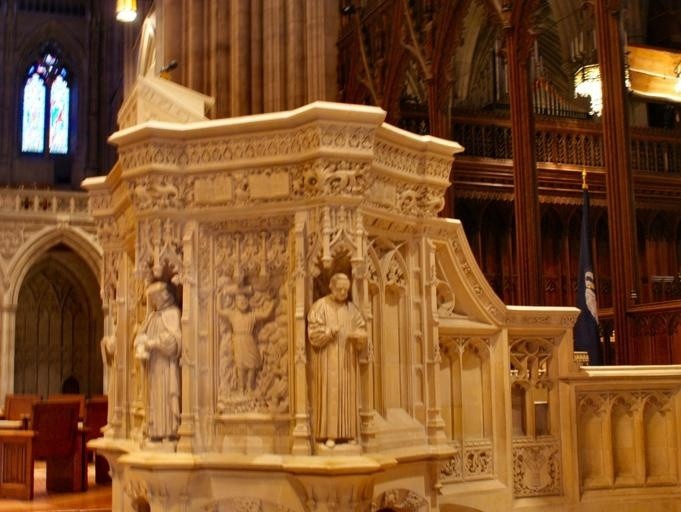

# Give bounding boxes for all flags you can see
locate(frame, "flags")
[572,184,603,365]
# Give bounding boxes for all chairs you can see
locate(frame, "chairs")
[0,393,110,512]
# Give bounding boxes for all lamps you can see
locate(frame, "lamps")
[571,61,630,117]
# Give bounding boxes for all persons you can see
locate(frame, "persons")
[214,292,278,395]
[306,272,368,445]
[135,281,181,438]
